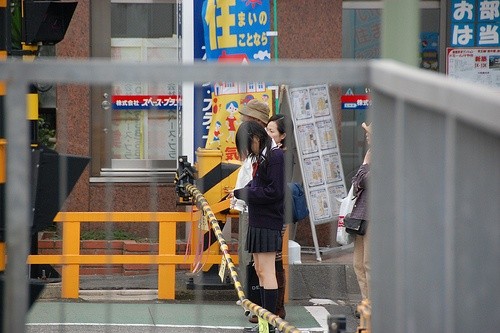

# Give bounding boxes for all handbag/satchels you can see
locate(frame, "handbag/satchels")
[343,214,368,236]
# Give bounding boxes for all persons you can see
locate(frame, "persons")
[344,119,376,333]
[222,110,296,332]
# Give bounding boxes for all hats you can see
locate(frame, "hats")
[238,98,270,123]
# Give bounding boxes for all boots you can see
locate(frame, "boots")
[244,286,279,332]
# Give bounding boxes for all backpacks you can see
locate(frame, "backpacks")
[284,180,309,223]
